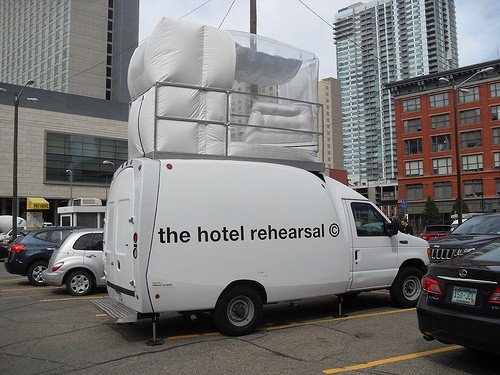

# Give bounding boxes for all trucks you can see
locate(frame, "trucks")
[90,156,434,338]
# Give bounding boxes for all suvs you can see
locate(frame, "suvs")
[427,212,500,264]
[4,226,92,286]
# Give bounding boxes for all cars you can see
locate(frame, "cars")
[42,227,107,296]
[0,241,9,258]
[415,236,500,355]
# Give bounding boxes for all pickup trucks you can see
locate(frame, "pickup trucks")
[420,224,452,240]
[0,226,28,241]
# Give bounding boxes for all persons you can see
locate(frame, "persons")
[400,219,413,235]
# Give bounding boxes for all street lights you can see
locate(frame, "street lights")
[0,79,38,235]
[66,169,74,206]
[438,65,494,227]
[103,159,116,175]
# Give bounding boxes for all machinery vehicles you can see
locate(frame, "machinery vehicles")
[0,214,53,234]
